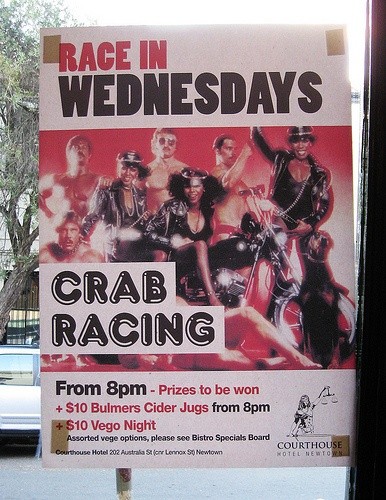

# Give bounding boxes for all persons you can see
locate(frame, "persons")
[38,126,332,369]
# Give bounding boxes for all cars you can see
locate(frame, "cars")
[0,345,40,445]
